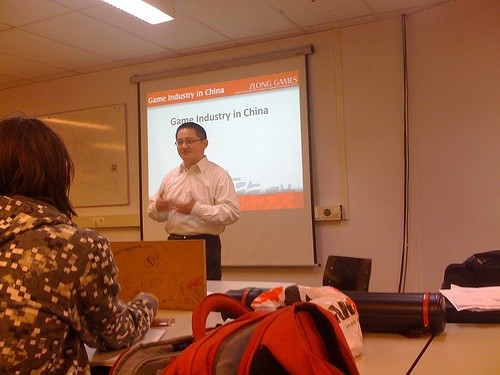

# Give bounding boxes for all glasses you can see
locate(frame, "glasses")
[175,139,202,145]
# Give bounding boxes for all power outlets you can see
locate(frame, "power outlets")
[93,218,105,225]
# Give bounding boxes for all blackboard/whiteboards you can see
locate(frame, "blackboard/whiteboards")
[35,103,129,208]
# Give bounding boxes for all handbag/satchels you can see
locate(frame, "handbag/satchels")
[251,286,364,356]
[112,293,359,375]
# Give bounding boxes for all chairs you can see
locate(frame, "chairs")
[442,251,499,293]
[322,255,372,291]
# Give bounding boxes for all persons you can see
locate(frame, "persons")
[148,122,240,280]
[0,117,159,375]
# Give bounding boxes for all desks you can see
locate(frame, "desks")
[86,280,500,373]
